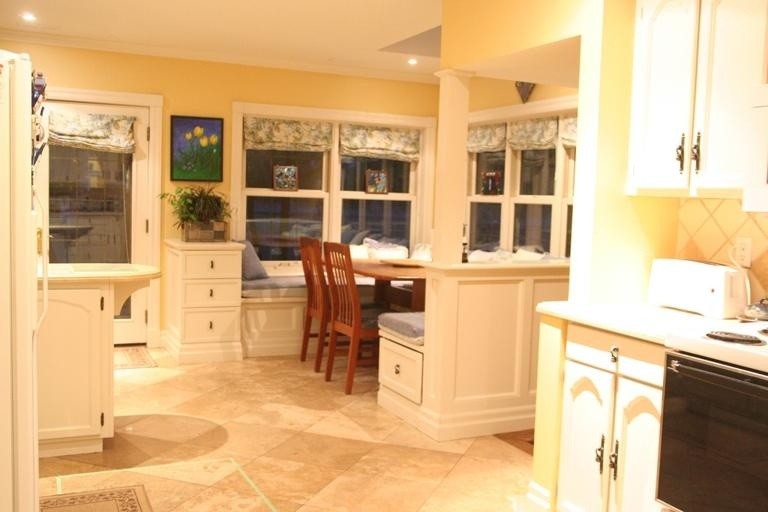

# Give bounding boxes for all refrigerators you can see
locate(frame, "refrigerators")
[1,56,48,512]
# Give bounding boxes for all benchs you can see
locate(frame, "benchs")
[241,274,412,357]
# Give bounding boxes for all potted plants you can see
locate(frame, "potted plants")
[162,185,229,243]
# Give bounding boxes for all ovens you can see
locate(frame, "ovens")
[655,350,767,510]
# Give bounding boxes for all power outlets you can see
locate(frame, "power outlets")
[736,237,752,269]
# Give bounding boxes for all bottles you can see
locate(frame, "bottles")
[462,224,468,264]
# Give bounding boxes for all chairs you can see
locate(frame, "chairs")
[324,243,399,394]
[298,237,383,373]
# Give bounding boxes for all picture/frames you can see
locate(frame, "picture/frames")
[273,165,299,191]
[365,169,389,193]
[481,171,501,194]
[170,114,224,181]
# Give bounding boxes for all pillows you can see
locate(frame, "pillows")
[467,248,561,264]
[231,240,269,281]
[332,235,433,279]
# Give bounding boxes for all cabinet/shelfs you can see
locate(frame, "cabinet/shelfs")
[533,307,668,512]
[159,239,241,366]
[624,0,768,211]
